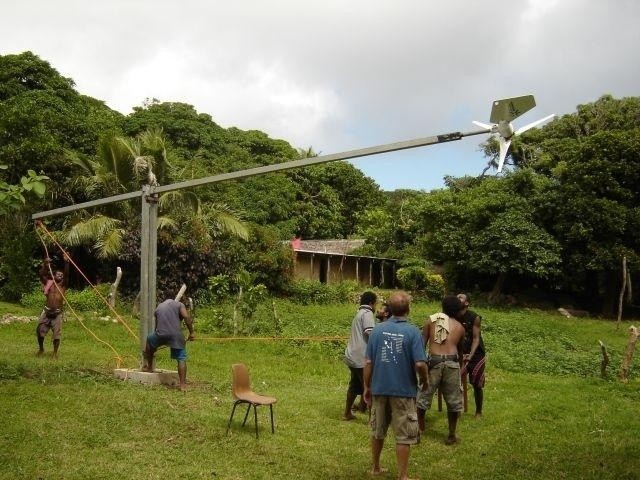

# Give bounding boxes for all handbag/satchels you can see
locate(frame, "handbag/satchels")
[45,311,57,319]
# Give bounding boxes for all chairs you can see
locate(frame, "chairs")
[227,363,278,439]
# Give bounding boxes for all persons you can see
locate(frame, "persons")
[352,303,392,412]
[417,296,465,444]
[362,291,430,480]
[142,290,197,389]
[454,292,486,418]
[342,292,377,428]
[36,251,70,358]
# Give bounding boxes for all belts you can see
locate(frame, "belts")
[431,354,460,359]
